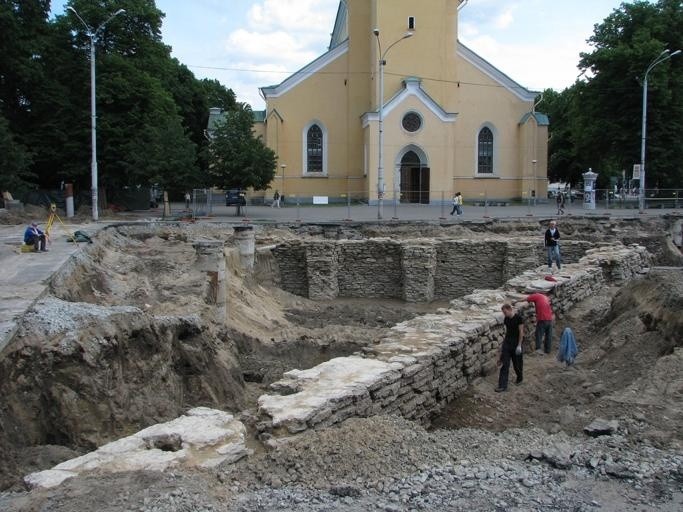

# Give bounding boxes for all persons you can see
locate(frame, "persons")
[557,191,565,214]
[270,189,282,208]
[24,221,48,254]
[184,190,192,207]
[457,193,462,213]
[494,304,524,392]
[450,193,459,216]
[527,291,553,353]
[543,220,561,269]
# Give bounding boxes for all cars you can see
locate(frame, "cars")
[225,188,247,206]
[548,190,621,199]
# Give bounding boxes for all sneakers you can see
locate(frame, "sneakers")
[516,377,522,385]
[495,387,506,392]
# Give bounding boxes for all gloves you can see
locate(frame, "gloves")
[516,346,521,355]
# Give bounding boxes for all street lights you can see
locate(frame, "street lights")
[66,5,126,222]
[373,28,413,219]
[638,48,681,214]
